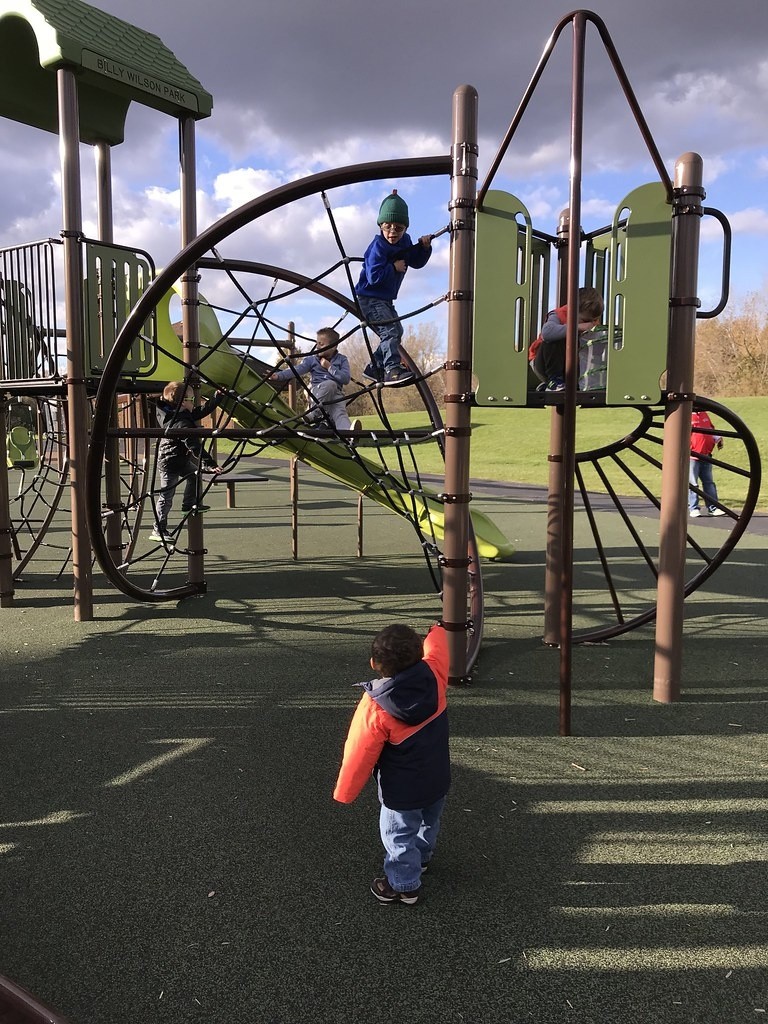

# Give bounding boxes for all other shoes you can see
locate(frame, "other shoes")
[304,414,314,423]
[535,382,547,392]
[707,505,726,516]
[544,379,565,392]
[350,419,362,447]
[689,509,702,517]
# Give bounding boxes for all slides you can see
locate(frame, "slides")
[115,263,518,560]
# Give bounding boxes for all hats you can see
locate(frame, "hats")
[377,189,410,228]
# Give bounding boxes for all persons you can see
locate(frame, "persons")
[354,189,432,386]
[687,412,726,517]
[148,381,227,544]
[529,286,605,393]
[263,326,362,449]
[333,622,452,907]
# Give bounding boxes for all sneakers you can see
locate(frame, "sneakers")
[420,862,429,874]
[370,876,420,905]
[149,529,175,542]
[362,364,382,382]
[181,503,211,513]
[382,367,414,386]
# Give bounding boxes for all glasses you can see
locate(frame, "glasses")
[183,397,195,404]
[380,224,404,233]
